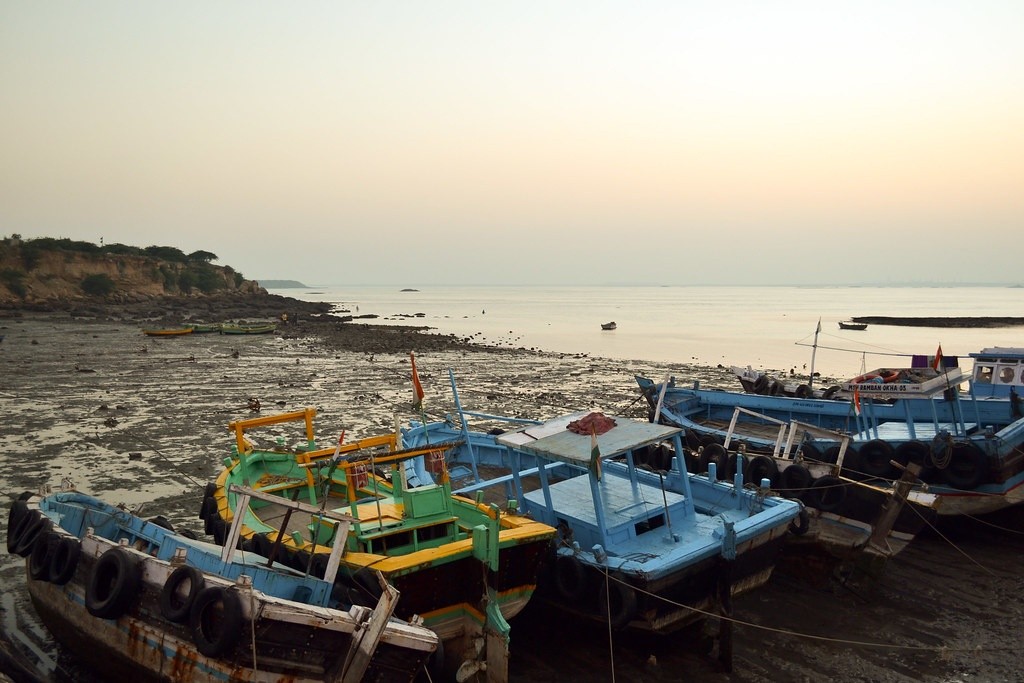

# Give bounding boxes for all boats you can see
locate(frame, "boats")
[601,322,617,330]
[838,321,869,331]
[496,407,933,586]
[221,322,277,335]
[142,324,195,336]
[632,367,1022,537]
[195,407,558,683]
[733,344,1023,428]
[181,322,221,332]
[393,349,800,642]
[9,483,438,683]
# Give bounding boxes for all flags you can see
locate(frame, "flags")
[410,354,426,413]
[816,321,821,335]
[933,346,943,375]
[324,429,345,487]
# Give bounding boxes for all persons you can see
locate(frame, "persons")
[282,313,297,324]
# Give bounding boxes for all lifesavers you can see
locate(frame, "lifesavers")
[851,375,884,385]
[877,370,900,382]
